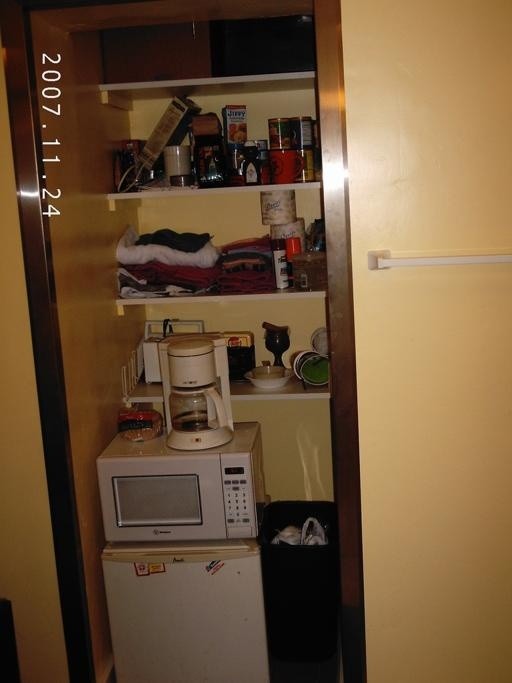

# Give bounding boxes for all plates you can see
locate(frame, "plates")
[244,368,295,389]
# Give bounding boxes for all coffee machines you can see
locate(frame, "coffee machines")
[161,339,235,453]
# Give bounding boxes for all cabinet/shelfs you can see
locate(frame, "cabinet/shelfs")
[100,70,330,403]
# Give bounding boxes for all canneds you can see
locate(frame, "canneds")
[266,115,321,184]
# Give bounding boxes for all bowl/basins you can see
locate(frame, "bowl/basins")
[255,365,285,378]
[292,327,329,387]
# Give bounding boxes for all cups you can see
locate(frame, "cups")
[267,148,306,184]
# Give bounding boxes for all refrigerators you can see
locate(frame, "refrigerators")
[98,542,271,683]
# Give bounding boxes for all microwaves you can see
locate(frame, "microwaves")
[96,420,269,545]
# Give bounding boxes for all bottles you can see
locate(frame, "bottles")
[242,138,261,185]
[272,235,303,291]
